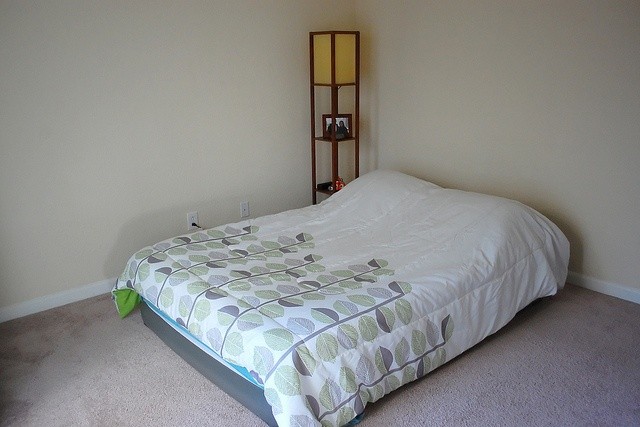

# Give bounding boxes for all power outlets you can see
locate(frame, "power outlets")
[184,212,199,231]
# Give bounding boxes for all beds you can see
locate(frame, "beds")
[111,168,571,427]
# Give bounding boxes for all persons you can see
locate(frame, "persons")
[328,118,338,131]
[338,120,348,132]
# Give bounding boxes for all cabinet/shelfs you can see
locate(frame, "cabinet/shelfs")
[310,31,359,203]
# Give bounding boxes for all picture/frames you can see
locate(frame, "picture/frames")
[323,114,353,138]
